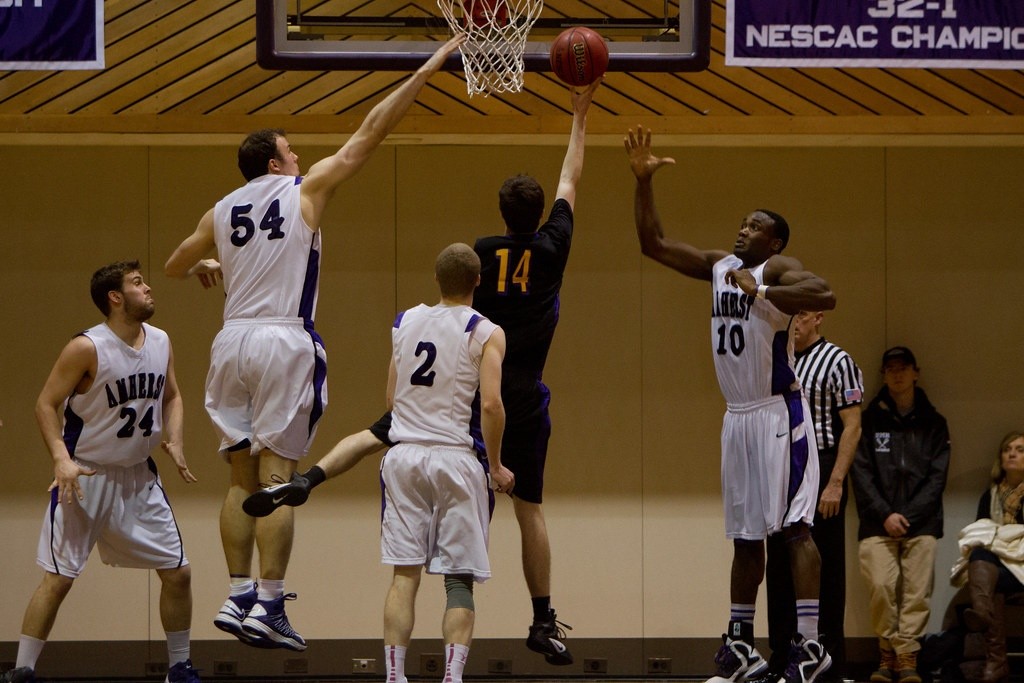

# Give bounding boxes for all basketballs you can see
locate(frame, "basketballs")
[550,25,610,85]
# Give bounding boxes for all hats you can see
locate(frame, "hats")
[881,346,915,362]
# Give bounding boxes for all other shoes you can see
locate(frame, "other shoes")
[165,658,204,683]
[893,652,922,683]
[0,666,36,683]
[870,651,895,683]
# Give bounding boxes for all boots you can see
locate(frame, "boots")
[972,593,1011,683]
[962,560,1000,639]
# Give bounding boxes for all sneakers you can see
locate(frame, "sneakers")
[777,629,833,683]
[240,592,309,651]
[705,632,768,683]
[213,577,284,648]
[241,471,312,517]
[525,607,575,666]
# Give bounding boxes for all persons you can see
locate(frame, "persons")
[851,343,952,683]
[766,308,864,683]
[376,240,518,683]
[241,72,608,669]
[2,258,208,683]
[964,428,1023,683]
[621,122,839,683]
[163,32,469,651]
